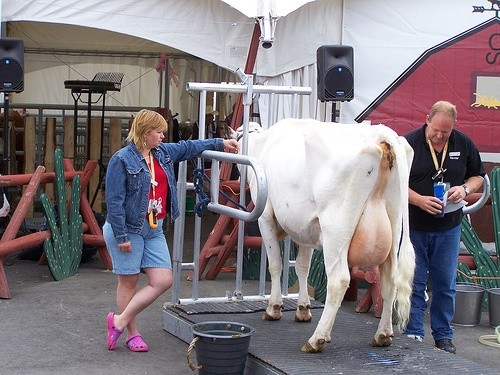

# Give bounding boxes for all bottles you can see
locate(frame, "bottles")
[433,185,444,217]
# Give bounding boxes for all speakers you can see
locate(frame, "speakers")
[317,45,354,103]
[0,38,24,93]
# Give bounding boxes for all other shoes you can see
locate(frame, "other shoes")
[435,338,457,354]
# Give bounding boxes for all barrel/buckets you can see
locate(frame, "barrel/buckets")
[191,321,254,375]
[487,288,500,328]
[452,285,485,326]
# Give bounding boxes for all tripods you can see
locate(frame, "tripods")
[0,92,32,235]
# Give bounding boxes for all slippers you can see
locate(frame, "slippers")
[124,333,150,352]
[106,311,125,350]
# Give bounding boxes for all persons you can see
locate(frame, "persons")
[189,105,219,140]
[102,109,241,352]
[403,100,487,354]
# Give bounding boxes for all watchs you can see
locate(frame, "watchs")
[462,184,471,195]
